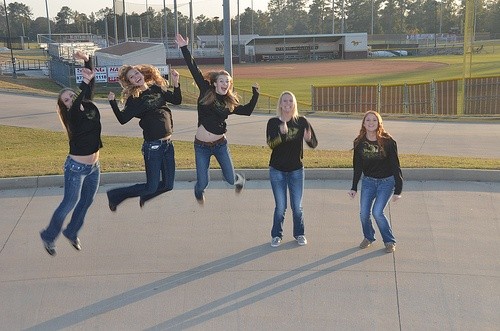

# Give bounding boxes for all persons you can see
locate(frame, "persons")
[106,63,183,214]
[265,90,318,247]
[38,49,103,256]
[175,33,260,207]
[348,110,403,253]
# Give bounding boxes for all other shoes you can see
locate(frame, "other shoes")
[61,231,83,252]
[234,171,245,195]
[194,187,205,207]
[40,231,56,256]
[139,198,144,206]
[106,190,117,211]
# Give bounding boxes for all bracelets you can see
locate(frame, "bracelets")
[84,77,91,81]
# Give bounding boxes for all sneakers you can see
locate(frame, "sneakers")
[360,238,372,248]
[385,241,396,252]
[295,235,307,245]
[271,236,281,247]
